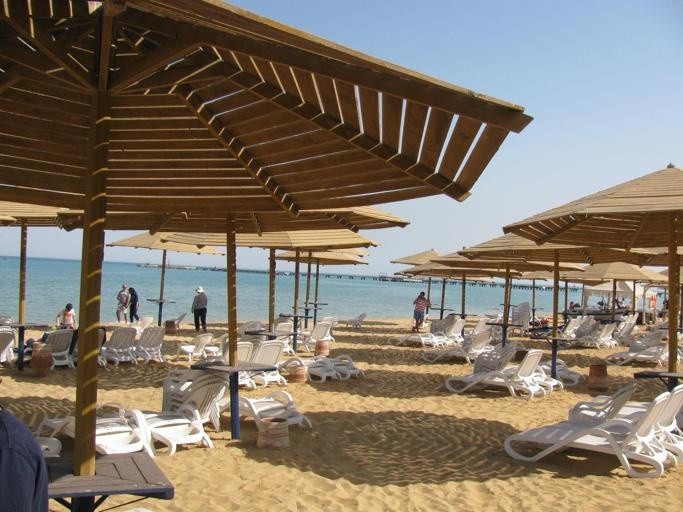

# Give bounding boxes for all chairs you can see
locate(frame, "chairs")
[388,301,682,480]
[1,303,369,510]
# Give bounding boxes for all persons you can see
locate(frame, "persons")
[1,409,49,512]
[116,284,140,324]
[23,302,77,353]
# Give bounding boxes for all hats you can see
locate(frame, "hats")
[195,287,205,293]
